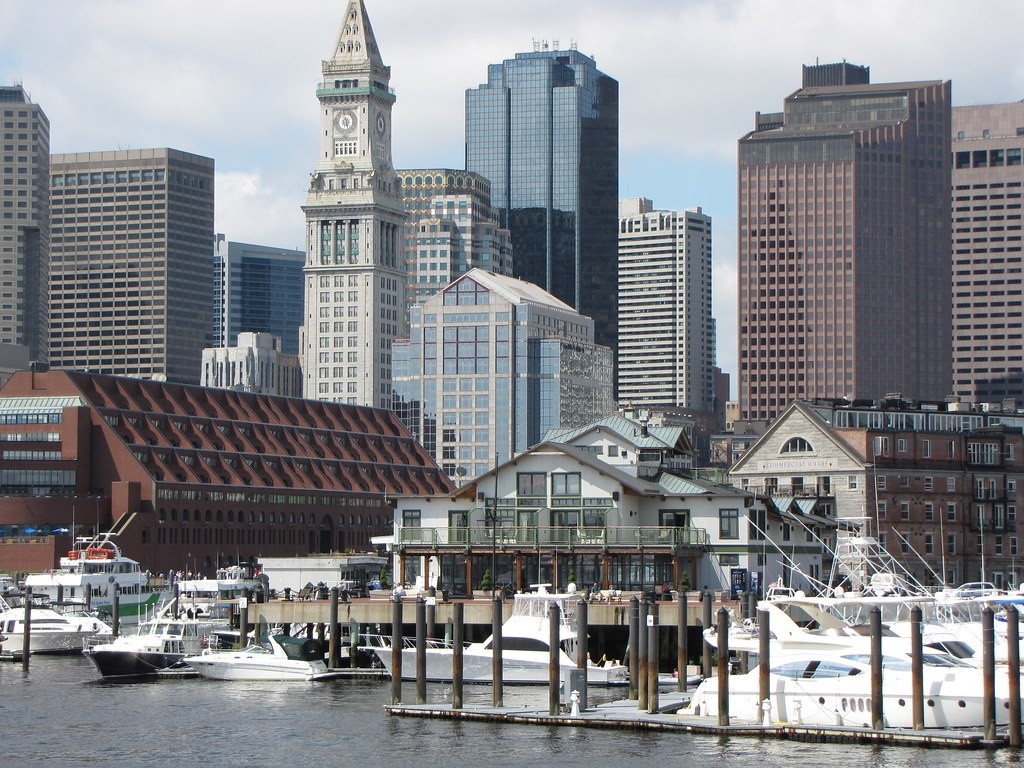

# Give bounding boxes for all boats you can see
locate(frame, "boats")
[357,544,628,684]
[676,440,1024,728]
[0,499,371,684]
[183,635,329,681]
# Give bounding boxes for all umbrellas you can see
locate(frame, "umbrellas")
[53,527,69,535]
[23,527,36,537]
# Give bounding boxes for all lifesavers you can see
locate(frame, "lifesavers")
[68,550,79,561]
[86,548,109,560]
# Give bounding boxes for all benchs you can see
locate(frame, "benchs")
[591,590,623,604]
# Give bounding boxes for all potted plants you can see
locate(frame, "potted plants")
[473,570,502,599]
[672,570,702,603]
[686,660,701,676]
[369,569,392,599]
[709,651,733,677]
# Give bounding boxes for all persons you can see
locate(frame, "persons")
[176,570,185,578]
[254,567,261,575]
[395,583,404,597]
[604,585,615,604]
[589,582,602,605]
[188,570,192,576]
[155,570,164,577]
[703,585,710,595]
[662,581,671,593]
[146,568,149,573]
